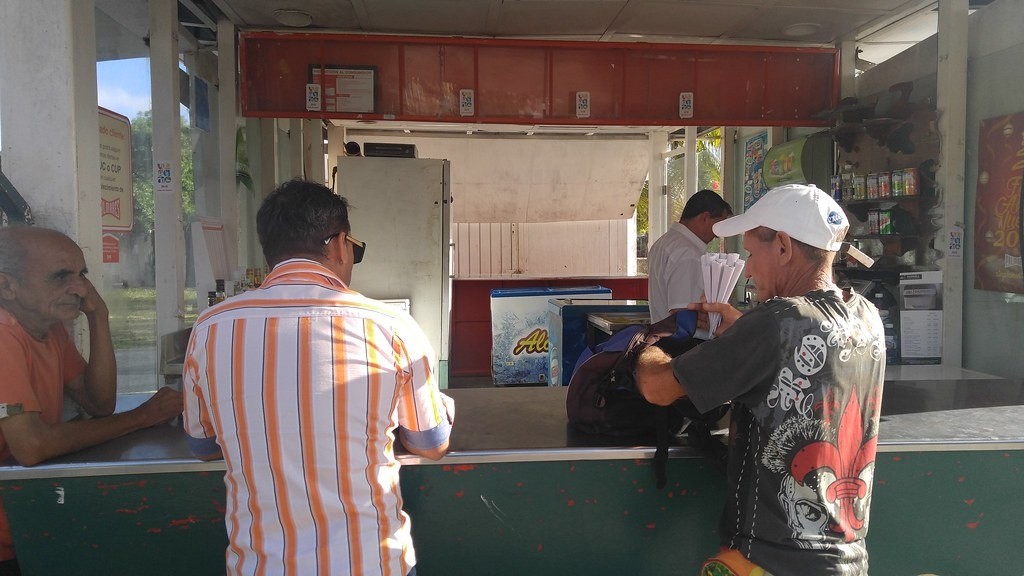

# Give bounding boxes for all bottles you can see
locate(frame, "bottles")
[207,265,268,308]
[842,164,855,198]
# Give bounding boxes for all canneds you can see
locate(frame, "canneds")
[829,168,920,201]
[867,207,896,235]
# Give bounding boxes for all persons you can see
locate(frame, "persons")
[636,182,886,576]
[182,179,455,576]
[0,225,184,576]
[647,189,734,341]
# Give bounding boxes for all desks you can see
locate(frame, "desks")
[586,312,650,350]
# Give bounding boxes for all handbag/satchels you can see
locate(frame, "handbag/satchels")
[566,309,731,490]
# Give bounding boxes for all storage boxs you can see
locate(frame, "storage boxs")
[867,210,880,234]
[899,271,942,365]
[879,210,896,235]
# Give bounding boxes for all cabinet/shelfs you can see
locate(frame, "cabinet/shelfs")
[833,193,933,273]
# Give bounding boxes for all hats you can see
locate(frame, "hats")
[712,184,875,268]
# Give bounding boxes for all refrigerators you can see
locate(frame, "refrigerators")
[336,154,456,390]
[489,287,649,388]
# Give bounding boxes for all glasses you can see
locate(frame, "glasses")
[323,232,366,265]
